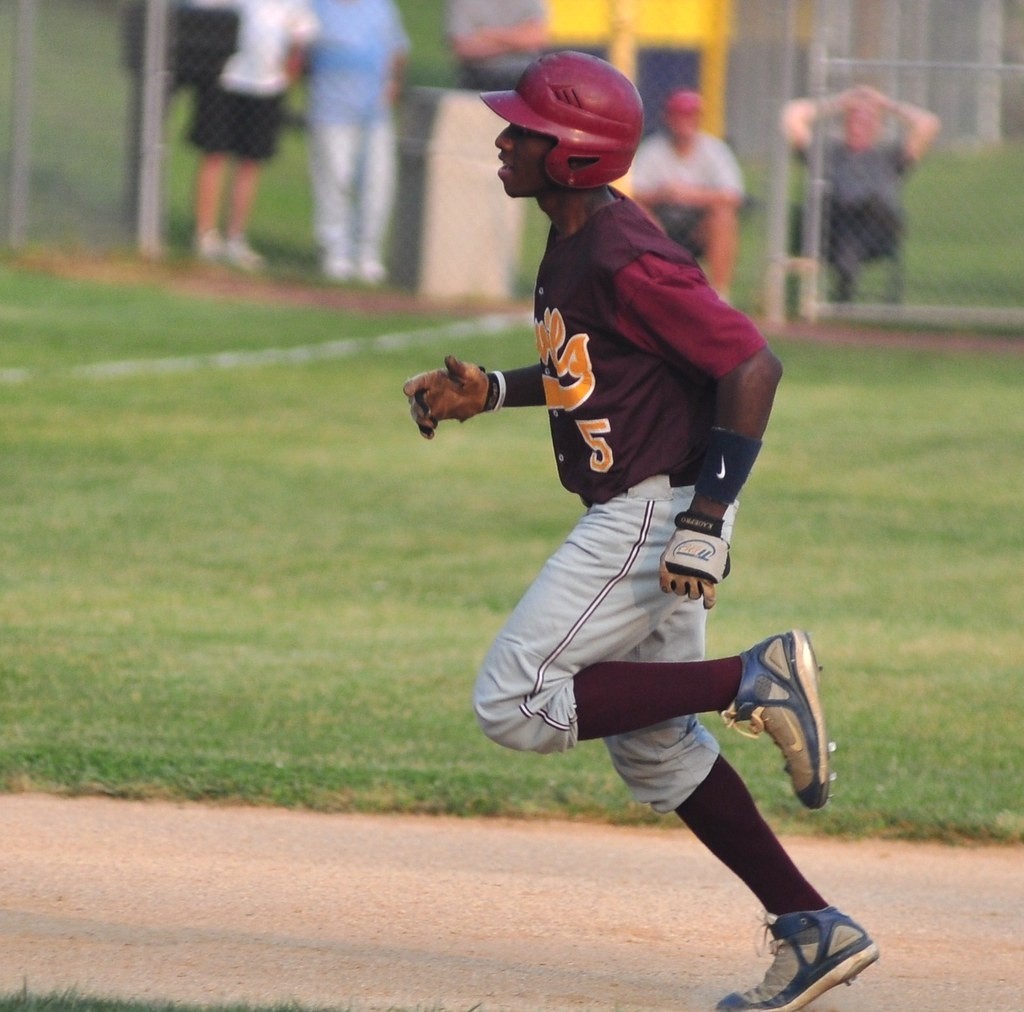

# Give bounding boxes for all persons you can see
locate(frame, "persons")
[302,1,412,288]
[775,85,939,304]
[447,0,556,87]
[404,51,880,1012]
[628,89,748,308]
[168,0,321,277]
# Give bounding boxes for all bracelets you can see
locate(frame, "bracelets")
[490,370,506,415]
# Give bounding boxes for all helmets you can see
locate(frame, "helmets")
[480,51,643,189]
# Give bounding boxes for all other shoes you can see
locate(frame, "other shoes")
[224,240,262,269]
[192,229,223,262]
[321,261,351,282]
[358,261,387,285]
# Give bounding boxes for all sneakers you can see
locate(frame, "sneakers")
[719,629,836,809]
[713,907,879,1012]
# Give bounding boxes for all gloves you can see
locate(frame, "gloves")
[404,355,500,440]
[660,511,730,610]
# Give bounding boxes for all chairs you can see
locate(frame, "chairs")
[785,206,907,302]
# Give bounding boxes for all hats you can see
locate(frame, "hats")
[665,92,699,116]
